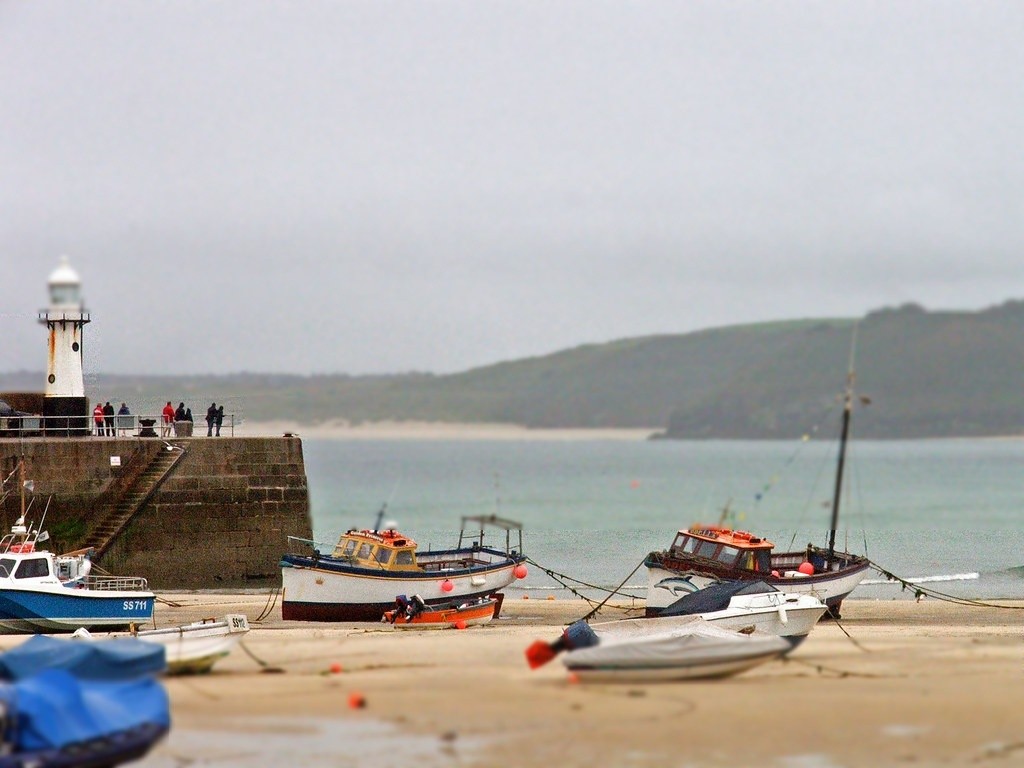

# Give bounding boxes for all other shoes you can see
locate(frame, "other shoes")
[216,434,220,436]
[107,435,110,436]
[113,434,115,436]
[98,433,104,436]
[207,434,212,437]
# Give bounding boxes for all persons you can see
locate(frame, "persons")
[117,402,130,421]
[93,403,104,436]
[162,401,193,437]
[103,401,115,437]
[206,402,225,437]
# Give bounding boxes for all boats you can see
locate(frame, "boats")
[381,593,497,629]
[524,616,792,684]
[620,579,829,655]
[0,495,157,638]
[276,514,528,623]
[643,372,871,620]
[0,639,173,768]
[0,613,253,678]
